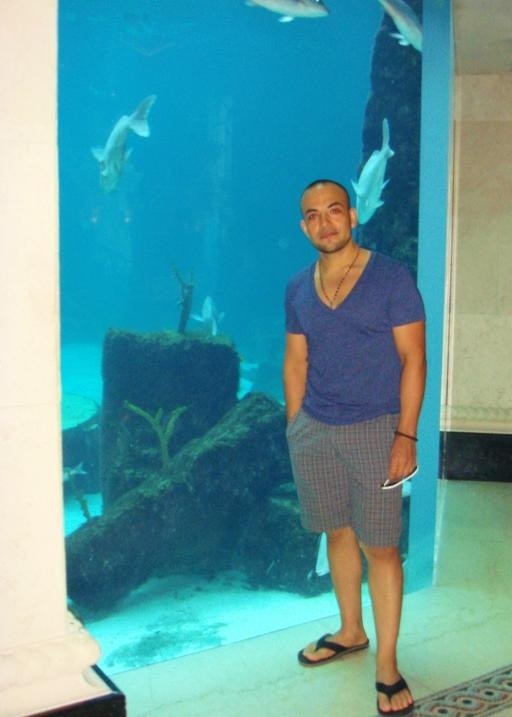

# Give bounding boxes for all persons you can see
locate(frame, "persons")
[283,180,427,716]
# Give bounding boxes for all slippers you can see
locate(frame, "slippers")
[297,633,369,668]
[376,673,413,714]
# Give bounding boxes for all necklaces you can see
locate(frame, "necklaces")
[318,246,360,311]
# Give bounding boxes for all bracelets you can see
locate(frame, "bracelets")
[394,430,420,444]
